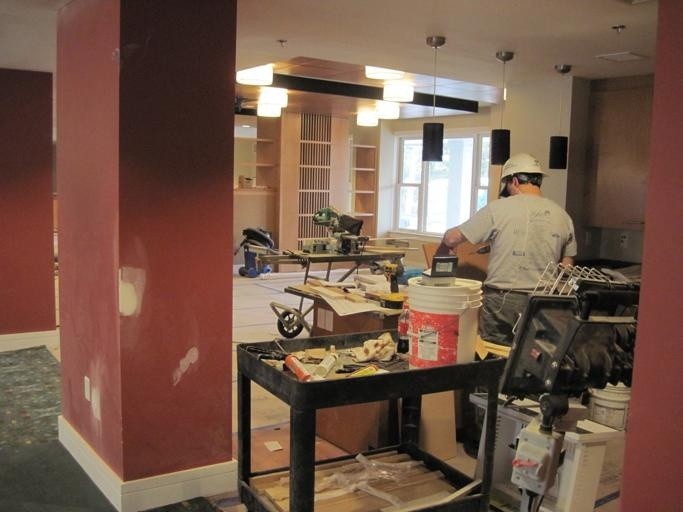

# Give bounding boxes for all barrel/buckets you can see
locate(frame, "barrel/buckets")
[409,276,484,370]
[578,386,631,431]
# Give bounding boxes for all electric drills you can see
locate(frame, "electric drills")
[375,257,408,309]
[469,245,490,255]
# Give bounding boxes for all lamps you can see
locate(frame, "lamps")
[490,50,514,165]
[548,64,572,169]
[356,66,415,128]
[422,36,445,162]
[237,63,289,117]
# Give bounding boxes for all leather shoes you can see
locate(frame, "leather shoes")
[463,437,479,458]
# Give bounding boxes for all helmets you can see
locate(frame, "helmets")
[501,154,550,182]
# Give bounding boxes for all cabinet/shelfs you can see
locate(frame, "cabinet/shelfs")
[238,329,507,512]
[232,111,381,273]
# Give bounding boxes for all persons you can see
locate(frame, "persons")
[436,152,579,460]
